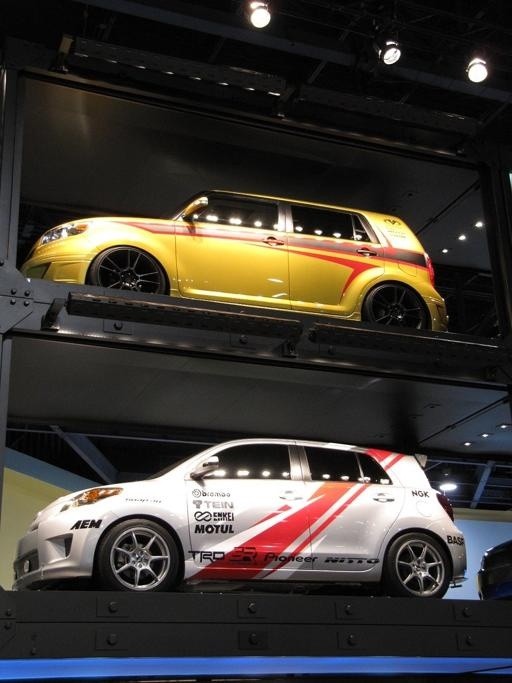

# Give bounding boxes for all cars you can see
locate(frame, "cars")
[21,187,451,332]
[13,435,471,599]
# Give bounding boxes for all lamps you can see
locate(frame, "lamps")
[246,0,272,29]
[466,57,488,84]
[371,32,401,66]
